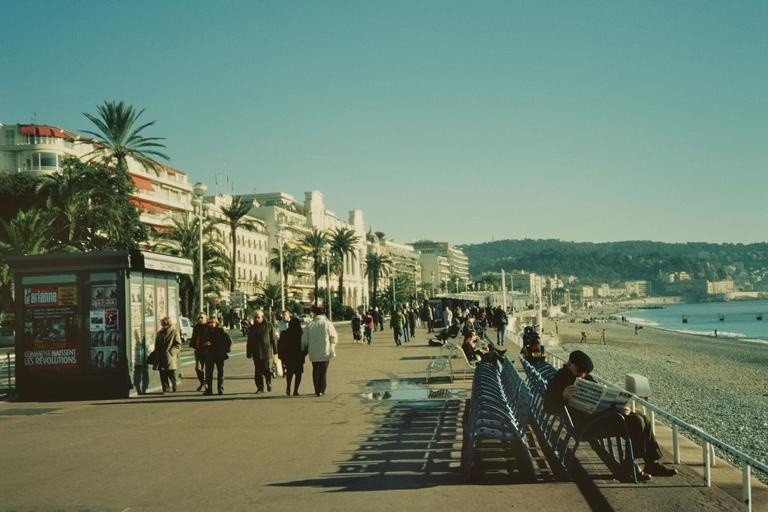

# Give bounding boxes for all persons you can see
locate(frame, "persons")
[634,324,639,335]
[580,330,588,343]
[91,331,105,347]
[246,308,279,394]
[90,351,104,368]
[602,329,608,345]
[351,299,515,368]
[107,333,117,346]
[277,317,309,396]
[715,328,719,338]
[105,351,118,369]
[543,350,678,481]
[306,306,338,396]
[277,309,291,336]
[201,316,232,396]
[152,317,183,396]
[188,312,209,392]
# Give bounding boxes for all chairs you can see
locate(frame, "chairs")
[463,356,637,484]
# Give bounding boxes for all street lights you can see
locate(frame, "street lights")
[390,260,495,312]
[322,241,333,323]
[273,228,292,314]
[189,181,209,318]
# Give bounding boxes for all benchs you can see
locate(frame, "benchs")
[426,321,478,383]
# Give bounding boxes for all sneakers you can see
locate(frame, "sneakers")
[293,392,299,396]
[255,383,272,393]
[286,388,290,396]
[645,461,677,476]
[315,391,326,396]
[629,462,651,480]
[197,382,223,395]
[162,385,177,395]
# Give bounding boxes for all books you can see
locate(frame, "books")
[567,377,633,416]
